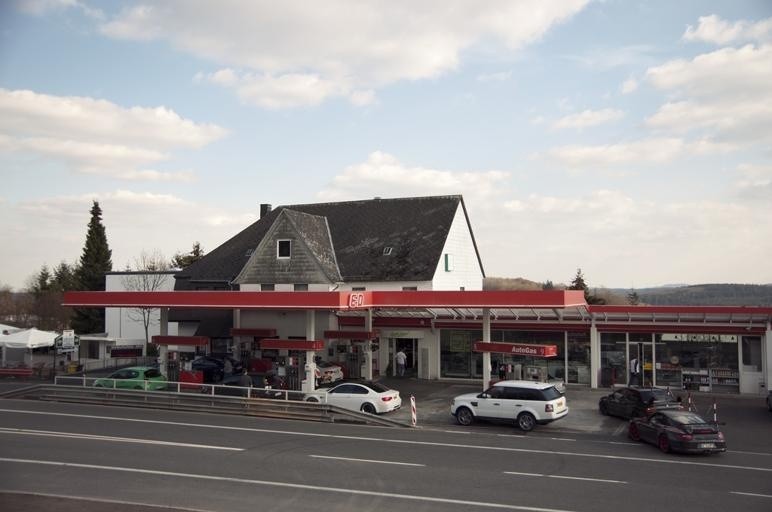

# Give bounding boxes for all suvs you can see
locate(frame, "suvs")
[450,378,570,433]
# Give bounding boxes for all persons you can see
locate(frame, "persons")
[223,356,233,378]
[628,356,642,388]
[644,356,652,388]
[395,349,407,376]
[239,367,252,407]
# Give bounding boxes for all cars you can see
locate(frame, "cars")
[275,359,345,385]
[598,381,684,422]
[199,370,290,401]
[303,378,402,417]
[92,365,170,398]
[627,408,728,457]
[193,351,244,376]
